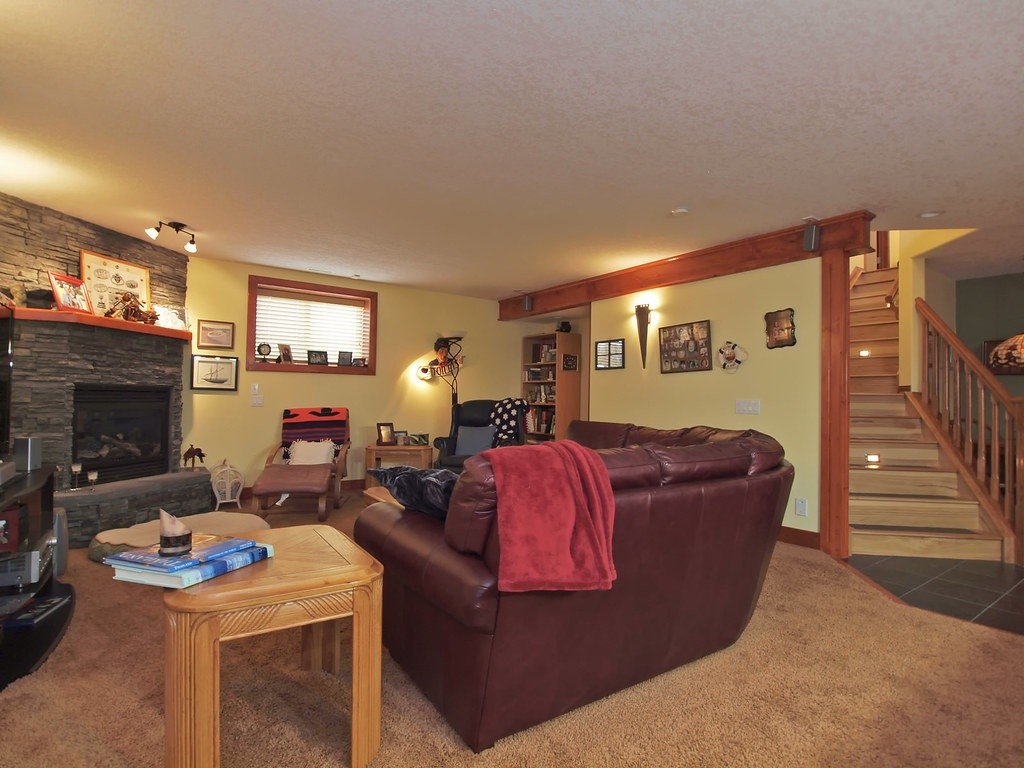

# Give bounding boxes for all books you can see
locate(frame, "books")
[527,440,548,444]
[522,343,556,434]
[102,532,276,589]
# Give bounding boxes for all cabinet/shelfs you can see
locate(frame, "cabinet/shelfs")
[0,464,77,695]
[521,332,582,443]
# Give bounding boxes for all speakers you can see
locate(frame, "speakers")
[803,226,820,251]
[15,436,42,472]
[524,295,531,311]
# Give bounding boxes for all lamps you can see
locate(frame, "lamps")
[636,303,651,367]
[416,337,465,405]
[988,333,1024,367]
[145,219,199,253]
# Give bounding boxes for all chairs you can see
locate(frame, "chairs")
[251,407,350,521]
[433,398,532,470]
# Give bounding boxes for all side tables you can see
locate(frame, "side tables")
[160,525,385,768]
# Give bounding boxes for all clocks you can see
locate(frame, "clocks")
[258,343,272,363]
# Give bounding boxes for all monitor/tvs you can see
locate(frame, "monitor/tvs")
[0,302,14,456]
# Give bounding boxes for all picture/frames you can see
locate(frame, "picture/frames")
[190,354,239,392]
[47,271,95,315]
[337,351,352,365]
[198,320,234,348]
[561,353,579,372]
[307,350,329,365]
[394,431,407,447]
[79,250,152,324]
[377,423,394,446]
[277,344,294,364]
[658,319,715,374]
[983,341,1024,375]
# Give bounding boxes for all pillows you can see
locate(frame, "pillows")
[455,425,496,457]
[289,439,336,465]
[365,467,467,517]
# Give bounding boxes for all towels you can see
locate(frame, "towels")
[481,438,619,591]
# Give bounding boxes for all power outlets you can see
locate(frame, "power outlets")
[796,499,807,515]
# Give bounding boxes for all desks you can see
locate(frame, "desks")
[365,447,434,503]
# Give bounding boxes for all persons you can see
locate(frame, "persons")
[9,285,28,308]
[61,285,83,309]
[662,321,709,371]
[282,347,292,361]
[311,353,326,364]
[429,337,453,366]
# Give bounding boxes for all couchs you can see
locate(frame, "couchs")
[352,420,794,754]
